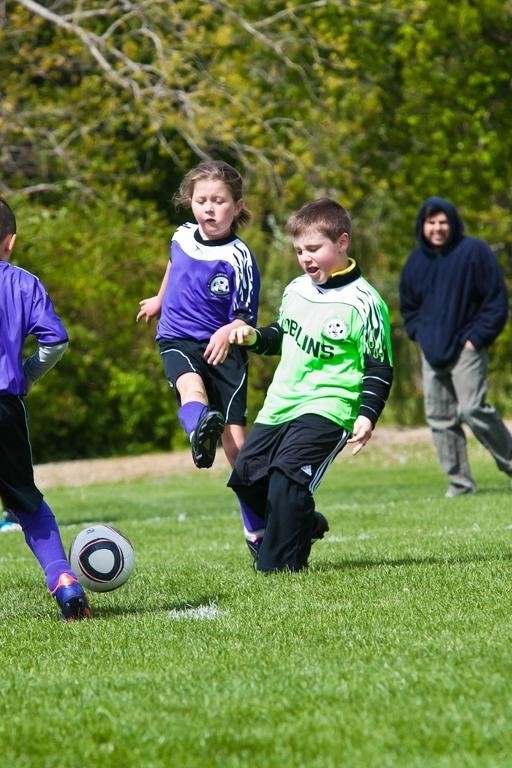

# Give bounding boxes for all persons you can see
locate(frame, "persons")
[130,156,262,468]
[398,197,510,499]
[222,193,394,576]
[0,193,95,620]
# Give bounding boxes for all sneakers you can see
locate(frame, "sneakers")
[310,513,328,545]
[48,572,93,618]
[244,538,261,563]
[188,413,224,468]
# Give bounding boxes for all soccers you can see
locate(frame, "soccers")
[68,525,135,593]
